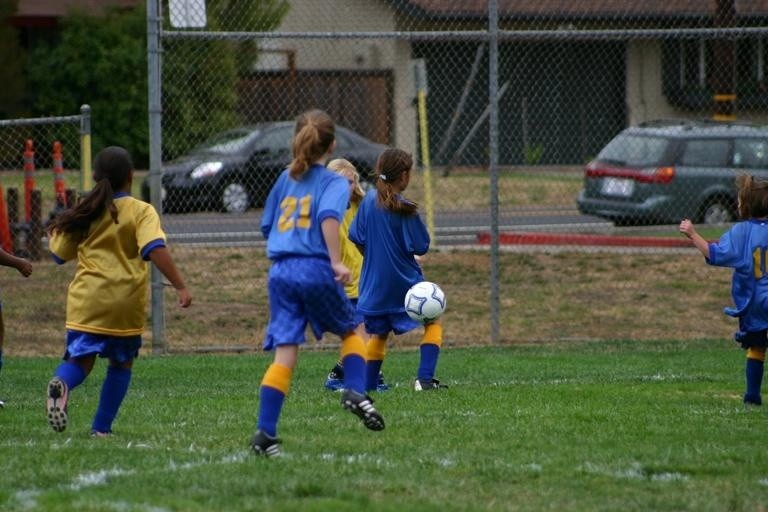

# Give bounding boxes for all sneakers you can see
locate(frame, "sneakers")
[743,391,763,407]
[90,429,116,439]
[414,378,450,392]
[46,375,69,434]
[248,427,285,460]
[339,387,386,431]
[376,371,388,392]
[324,373,346,390]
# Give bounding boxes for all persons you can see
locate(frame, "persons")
[0,245,33,410]
[318,160,394,394]
[349,147,450,395]
[246,108,389,467]
[43,143,193,438]
[679,178,768,404]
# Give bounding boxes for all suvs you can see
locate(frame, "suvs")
[577,117,766,231]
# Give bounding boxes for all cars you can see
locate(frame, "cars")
[141,121,394,216]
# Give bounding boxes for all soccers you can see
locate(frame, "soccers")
[405,281,446,323]
